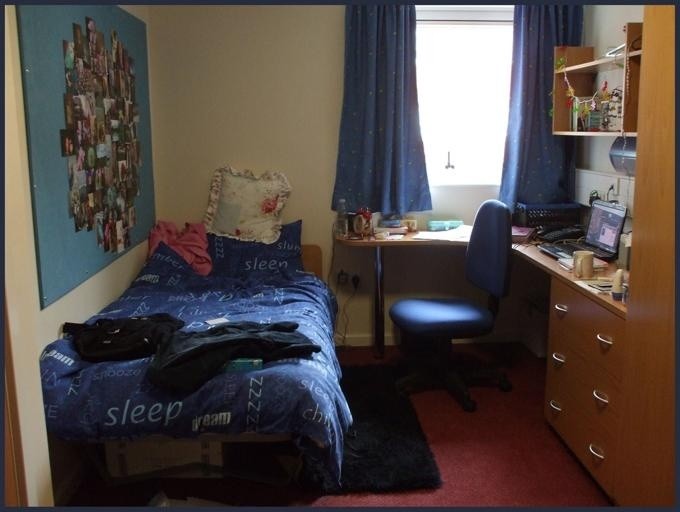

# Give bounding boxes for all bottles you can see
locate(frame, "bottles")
[335,198,349,239]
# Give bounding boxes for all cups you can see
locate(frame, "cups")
[572,249,596,281]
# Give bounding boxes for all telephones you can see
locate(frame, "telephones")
[538,224,585,242]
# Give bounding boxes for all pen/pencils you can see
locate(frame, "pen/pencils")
[599,285,613,288]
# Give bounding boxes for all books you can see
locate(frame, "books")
[557,257,608,273]
[569,95,599,131]
[374,227,408,234]
[512,225,535,244]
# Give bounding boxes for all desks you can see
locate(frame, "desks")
[330,224,628,503]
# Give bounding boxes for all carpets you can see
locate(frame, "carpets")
[327,360,443,496]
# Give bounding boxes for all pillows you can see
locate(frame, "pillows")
[201,166,304,278]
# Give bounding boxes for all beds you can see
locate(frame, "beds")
[41,243,344,508]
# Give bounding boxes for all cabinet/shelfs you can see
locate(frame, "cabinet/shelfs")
[551,21,641,138]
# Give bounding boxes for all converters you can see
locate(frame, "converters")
[337,272,348,285]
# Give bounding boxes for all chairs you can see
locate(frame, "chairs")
[389,199,518,413]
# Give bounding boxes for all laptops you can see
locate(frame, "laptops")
[536,199,628,261]
